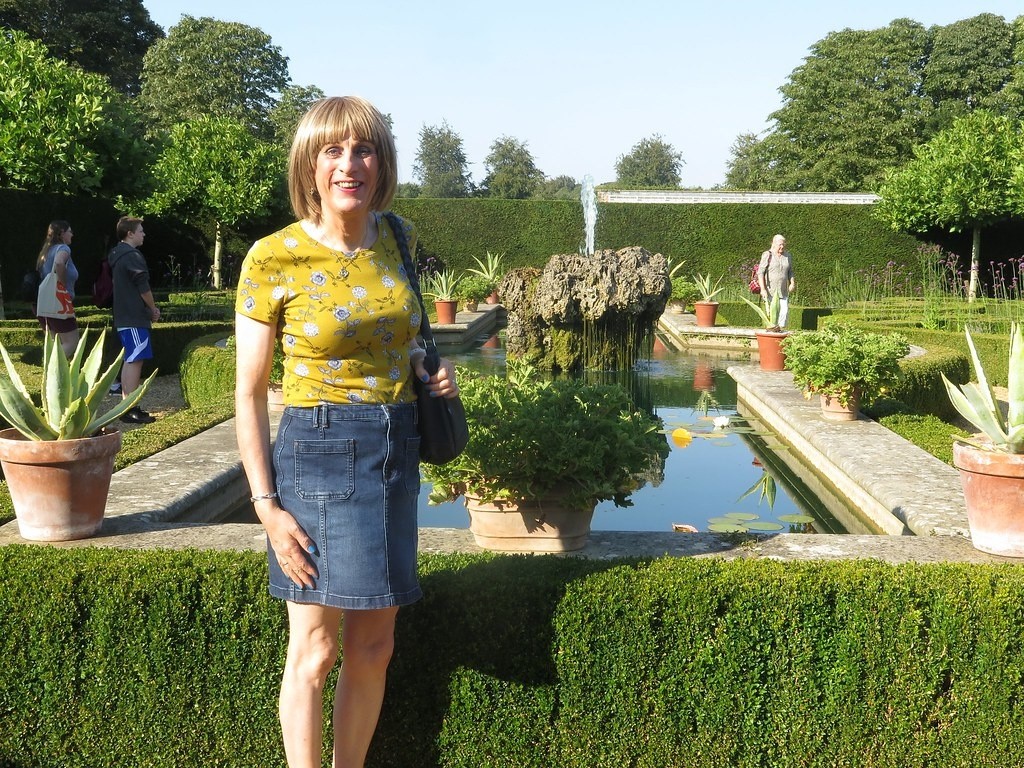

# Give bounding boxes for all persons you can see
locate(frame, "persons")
[36,220,78,359]
[104,229,122,396]
[108,217,160,424]
[757,234,795,329]
[235,96,460,768]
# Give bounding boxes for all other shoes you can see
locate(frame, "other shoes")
[108,385,123,396]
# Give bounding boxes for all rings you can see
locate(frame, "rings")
[283,562,288,566]
[453,380,456,384]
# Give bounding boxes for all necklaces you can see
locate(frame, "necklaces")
[322,221,369,277]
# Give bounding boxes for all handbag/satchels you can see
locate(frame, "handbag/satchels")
[37,245,75,319]
[749,251,772,294]
[90,257,114,309]
[413,340,469,465]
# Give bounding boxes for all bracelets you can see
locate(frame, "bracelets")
[250,493,277,503]
[409,347,426,355]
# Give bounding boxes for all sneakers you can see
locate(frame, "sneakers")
[120,407,155,423]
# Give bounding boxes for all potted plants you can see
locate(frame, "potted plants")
[779,321,911,422]
[460,274,497,312]
[0,319,159,542]
[739,290,790,371]
[419,366,672,554]
[693,364,722,417]
[666,276,694,313]
[421,268,465,324]
[735,457,777,512]
[465,251,506,304]
[692,272,726,327]
[940,319,1024,559]
